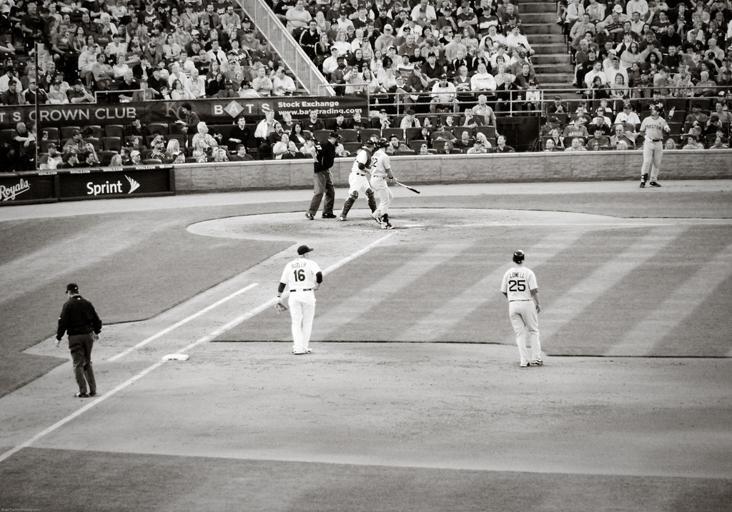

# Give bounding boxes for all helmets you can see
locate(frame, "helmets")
[513,249,525,264]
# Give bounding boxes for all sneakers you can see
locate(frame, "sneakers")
[372,213,381,224]
[650,182,661,187]
[640,173,648,187]
[531,360,543,366]
[304,213,314,220]
[75,391,89,398]
[322,212,337,218]
[339,212,347,221]
[380,221,395,229]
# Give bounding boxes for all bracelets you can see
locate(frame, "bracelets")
[388,176,393,180]
[277,295,281,298]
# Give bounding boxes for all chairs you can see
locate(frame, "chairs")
[1,106,716,172]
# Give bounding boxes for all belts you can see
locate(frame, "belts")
[654,139,662,141]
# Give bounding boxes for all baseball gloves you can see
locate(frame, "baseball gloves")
[275,303,287,311]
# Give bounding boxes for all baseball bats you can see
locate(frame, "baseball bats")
[397,182,420,194]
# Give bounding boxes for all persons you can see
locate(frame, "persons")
[275,245,323,354]
[2,1,732,168]
[306,132,336,220]
[639,105,672,187]
[339,139,376,221]
[369,138,398,230]
[54,281,103,398]
[500,250,545,368]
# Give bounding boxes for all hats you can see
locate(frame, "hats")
[296,245,313,255]
[65,283,79,293]
